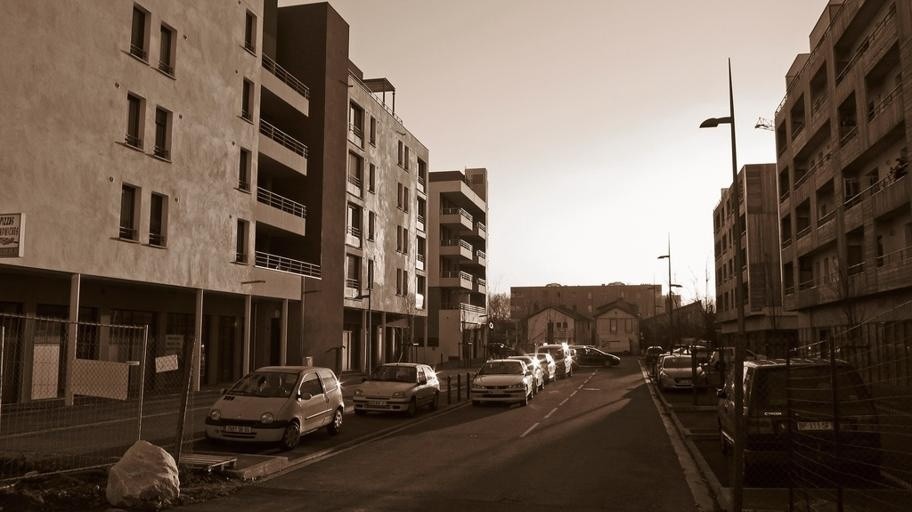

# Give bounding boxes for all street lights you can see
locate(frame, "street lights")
[657,230,684,343]
[698,57,746,348]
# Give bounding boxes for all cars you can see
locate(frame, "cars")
[715,357,880,482]
[576,344,620,367]
[353,362,440,417]
[710,347,768,371]
[471,342,577,407]
[204,366,345,451]
[645,338,712,392]
